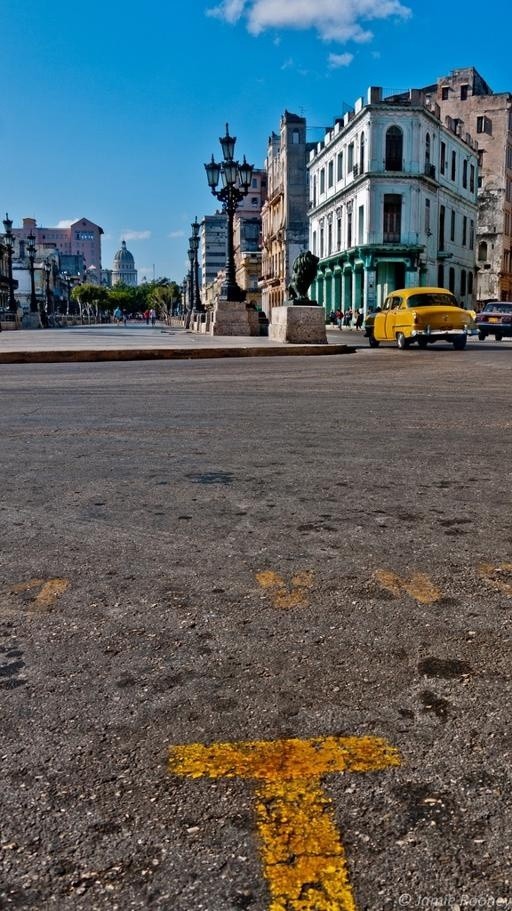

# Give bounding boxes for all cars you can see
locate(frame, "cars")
[363,287,474,349]
[475,301,512,340]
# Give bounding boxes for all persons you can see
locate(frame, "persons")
[329,306,373,331]
[102,307,157,325]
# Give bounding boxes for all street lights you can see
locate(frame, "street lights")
[26,228,38,313]
[183,214,206,313]
[44,257,51,315]
[2,212,18,321]
[65,271,71,316]
[203,122,255,301]
